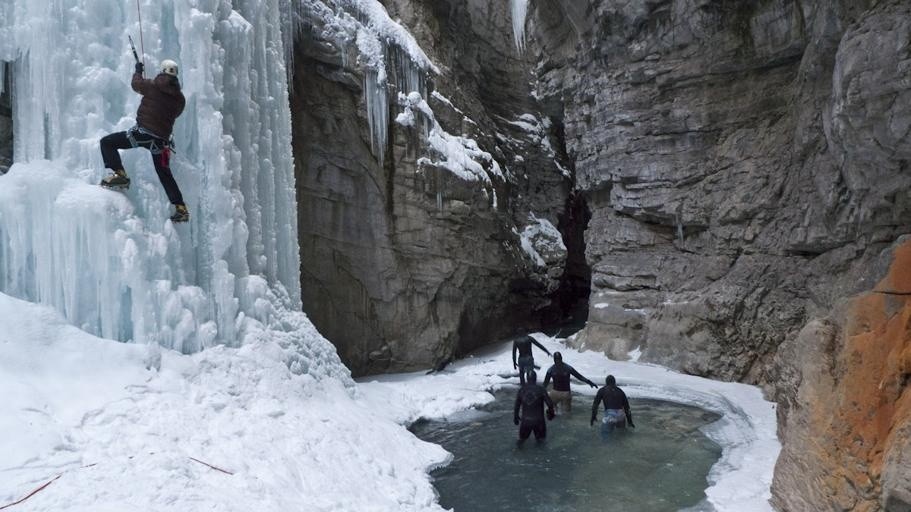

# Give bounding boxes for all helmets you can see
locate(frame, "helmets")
[161,61,178,76]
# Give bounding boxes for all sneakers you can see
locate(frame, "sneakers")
[170,210,189,218]
[102,173,130,184]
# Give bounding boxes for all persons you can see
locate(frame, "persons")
[513,369,556,446]
[512,327,552,387]
[539,352,598,416]
[99,59,189,221]
[590,374,635,441]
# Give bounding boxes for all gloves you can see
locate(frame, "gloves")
[591,417,598,425]
[514,361,518,369]
[136,61,144,73]
[588,382,598,388]
[514,414,521,425]
